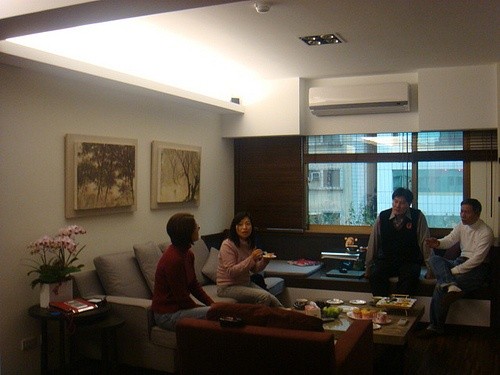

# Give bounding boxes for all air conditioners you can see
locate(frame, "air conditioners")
[308,82,410,116]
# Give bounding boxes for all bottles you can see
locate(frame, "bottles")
[304,301,320,319]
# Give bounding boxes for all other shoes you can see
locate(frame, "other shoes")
[417,329,445,339]
[447,286,462,294]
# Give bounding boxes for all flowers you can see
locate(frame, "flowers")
[27,225,87,288]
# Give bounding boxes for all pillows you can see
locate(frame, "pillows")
[161,236,207,285]
[202,246,219,282]
[134,240,162,292]
[207,301,324,331]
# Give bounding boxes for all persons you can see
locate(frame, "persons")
[365,188,435,298]
[152,214,214,327]
[425,198,494,346]
[217,212,285,307]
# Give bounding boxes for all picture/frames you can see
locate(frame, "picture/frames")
[151,141,202,210]
[65,132,138,219]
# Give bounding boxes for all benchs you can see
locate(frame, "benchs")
[201,229,500,328]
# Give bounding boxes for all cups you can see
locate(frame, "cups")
[292,299,308,311]
[352,307,387,322]
[397,297,411,307]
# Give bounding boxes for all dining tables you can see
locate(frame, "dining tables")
[289,298,426,346]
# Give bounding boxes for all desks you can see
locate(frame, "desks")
[28,303,127,375]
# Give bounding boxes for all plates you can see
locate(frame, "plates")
[338,305,353,313]
[370,318,392,324]
[349,301,367,304]
[327,299,344,304]
[347,312,372,320]
[261,254,276,258]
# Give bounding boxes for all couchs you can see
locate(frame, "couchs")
[176,300,376,375]
[69,237,283,375]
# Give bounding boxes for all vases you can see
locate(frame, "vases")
[40,279,74,308]
